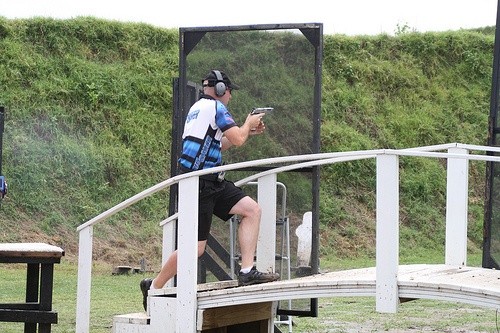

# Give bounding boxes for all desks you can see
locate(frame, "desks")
[0,242,65,333]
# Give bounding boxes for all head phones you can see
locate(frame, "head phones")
[211,69,226,97]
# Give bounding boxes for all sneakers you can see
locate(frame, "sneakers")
[239,266,279,285]
[140,278,155,312]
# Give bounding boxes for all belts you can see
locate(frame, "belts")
[180,167,226,182]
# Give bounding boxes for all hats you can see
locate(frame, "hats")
[202,71,241,90]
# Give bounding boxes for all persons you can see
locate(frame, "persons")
[140,69,280,312]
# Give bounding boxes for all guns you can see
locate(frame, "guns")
[250,107,274,130]
